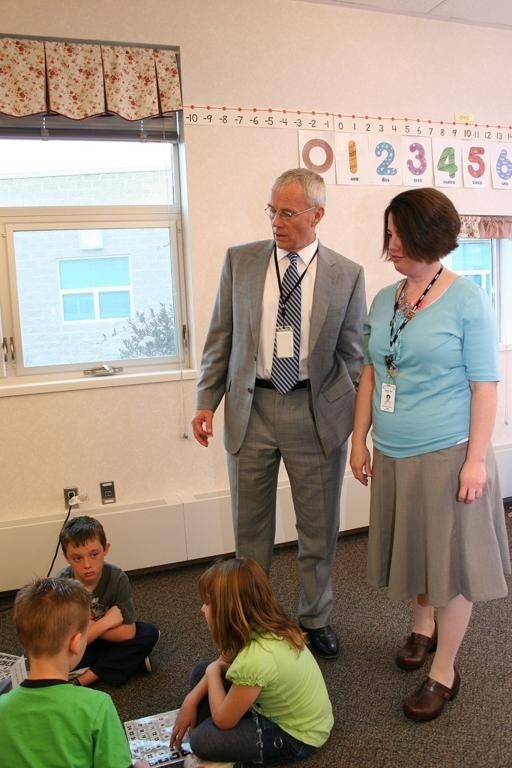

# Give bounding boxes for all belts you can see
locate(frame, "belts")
[255,378,310,390]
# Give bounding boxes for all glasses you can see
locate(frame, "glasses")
[264,204,318,219]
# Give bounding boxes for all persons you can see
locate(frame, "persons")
[0,574,141,768]
[192,168,370,661]
[351,182,511,723]
[168,554,337,762]
[57,511,160,687]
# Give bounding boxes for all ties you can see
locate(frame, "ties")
[270,253,302,398]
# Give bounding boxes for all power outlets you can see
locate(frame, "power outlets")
[63,487,79,510]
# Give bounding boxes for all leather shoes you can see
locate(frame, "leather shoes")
[298,622,339,659]
[403,667,459,721]
[397,621,438,669]
